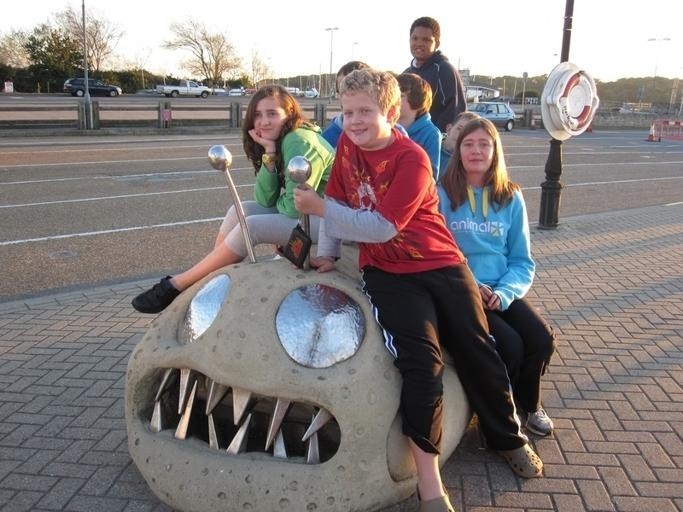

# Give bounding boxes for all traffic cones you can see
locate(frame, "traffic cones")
[646,120,657,141]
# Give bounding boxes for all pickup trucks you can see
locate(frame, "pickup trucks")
[155,80,212,98]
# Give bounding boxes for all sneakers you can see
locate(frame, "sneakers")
[518,406,554,436]
[131,275,181,314]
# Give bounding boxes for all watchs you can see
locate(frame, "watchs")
[262,152,279,164]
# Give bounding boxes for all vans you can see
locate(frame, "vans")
[62,78,122,97]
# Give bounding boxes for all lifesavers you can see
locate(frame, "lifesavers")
[562,75,593,130]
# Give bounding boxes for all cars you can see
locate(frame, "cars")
[214,86,318,96]
[466,101,515,131]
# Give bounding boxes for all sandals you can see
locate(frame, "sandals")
[498,440,544,478]
[415,479,455,512]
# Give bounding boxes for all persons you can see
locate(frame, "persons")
[132,84,336,313]
[440,112,480,178]
[394,73,442,182]
[320,61,370,151]
[402,17,466,133]
[437,118,554,437]
[292,66,543,512]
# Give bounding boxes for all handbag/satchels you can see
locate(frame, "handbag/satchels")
[278,223,312,269]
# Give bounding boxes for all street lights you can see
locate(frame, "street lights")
[647,37,673,91]
[324,27,338,105]
